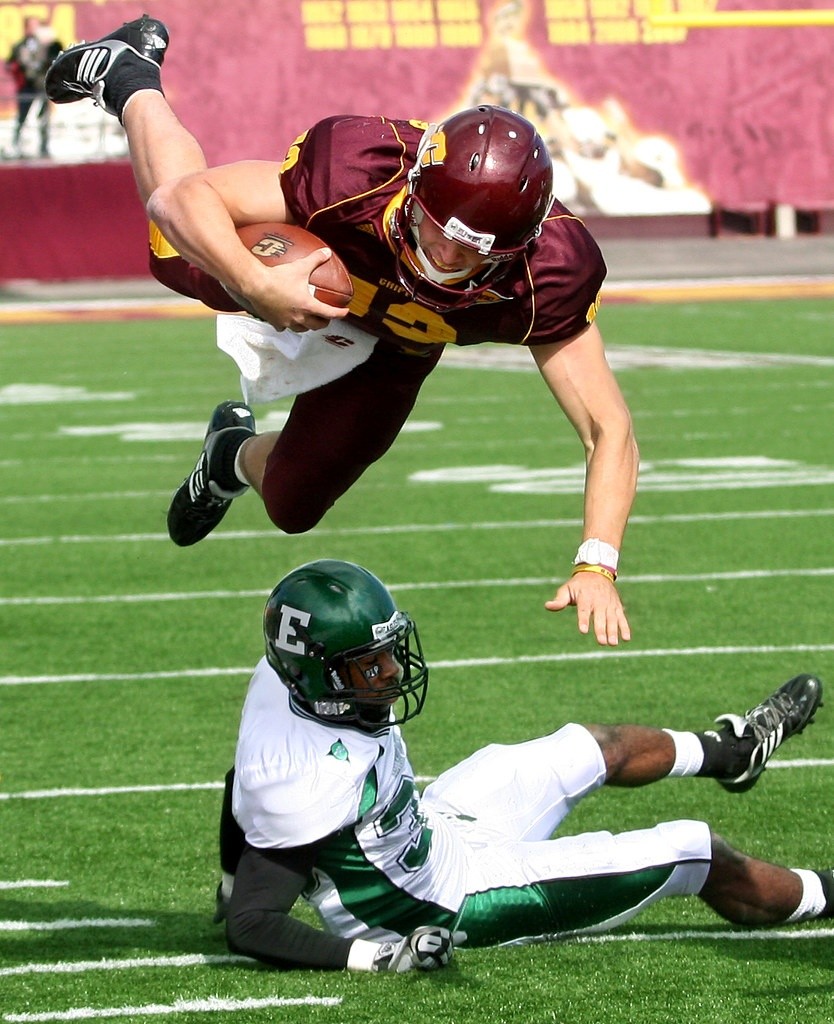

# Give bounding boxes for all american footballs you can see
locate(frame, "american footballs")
[235,224,356,311]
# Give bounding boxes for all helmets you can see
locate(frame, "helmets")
[388,105,554,310]
[263,559,427,732]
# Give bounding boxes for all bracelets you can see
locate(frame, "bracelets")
[571,539,619,583]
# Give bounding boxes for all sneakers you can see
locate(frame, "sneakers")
[810,866,834,920]
[168,401,256,547]
[45,13,172,117]
[714,673,823,794]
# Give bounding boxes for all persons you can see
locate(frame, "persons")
[213,560,834,972]
[46,15,634,648]
[7,17,53,158]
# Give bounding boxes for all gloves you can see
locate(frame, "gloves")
[371,926,467,972]
